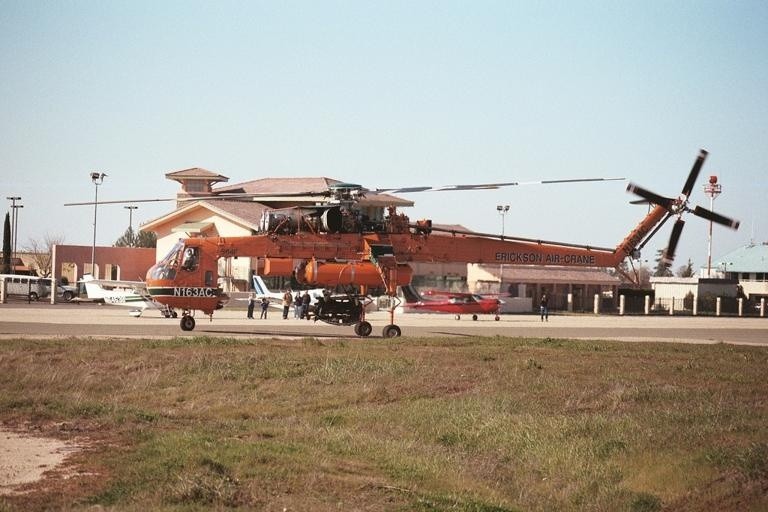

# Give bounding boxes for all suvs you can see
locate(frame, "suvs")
[42,277,79,301]
[0,256,37,276]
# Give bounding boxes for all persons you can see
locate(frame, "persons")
[247,288,311,319]
[540,294,549,322]
[184,249,193,269]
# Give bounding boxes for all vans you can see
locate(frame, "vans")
[0,273,44,302]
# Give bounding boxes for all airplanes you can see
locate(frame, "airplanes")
[76,272,179,318]
[395,283,513,323]
[235,274,374,318]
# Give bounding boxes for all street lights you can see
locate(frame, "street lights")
[700,176,724,279]
[122,204,138,246]
[10,205,24,274]
[5,196,21,274]
[719,261,733,279]
[496,204,512,238]
[89,171,108,280]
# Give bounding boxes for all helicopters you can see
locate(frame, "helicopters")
[62,143,741,342]
